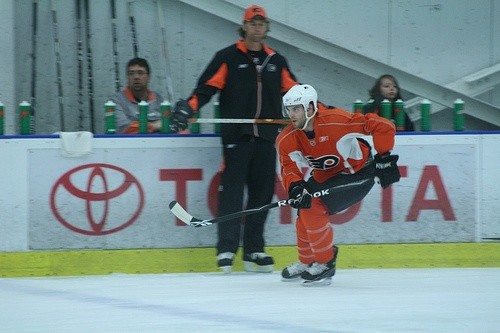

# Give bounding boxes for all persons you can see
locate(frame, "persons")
[275,84,400,287]
[363,74,415,132]
[112,59,187,134]
[169,5,329,274]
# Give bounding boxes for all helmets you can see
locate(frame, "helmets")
[282,84,318,112]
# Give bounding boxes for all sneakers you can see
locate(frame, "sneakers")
[242,251,273,272]
[215,252,234,273]
[301,246,338,286]
[282,260,314,282]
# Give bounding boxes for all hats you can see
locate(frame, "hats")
[244,5,266,21]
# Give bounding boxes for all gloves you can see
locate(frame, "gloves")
[289,180,312,209]
[168,97,193,132]
[372,152,400,187]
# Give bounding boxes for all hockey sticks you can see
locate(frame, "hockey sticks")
[187,118,293,125]
[169,175,381,227]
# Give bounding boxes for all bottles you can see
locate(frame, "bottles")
[159,99,175,134]
[420,98,432,131]
[18,100,34,135]
[353,97,365,114]
[104,100,118,134]
[392,98,407,132]
[212,98,223,133]
[136,101,149,134]
[187,108,200,135]
[0,103,5,136]
[380,98,394,124]
[454,98,466,130]
